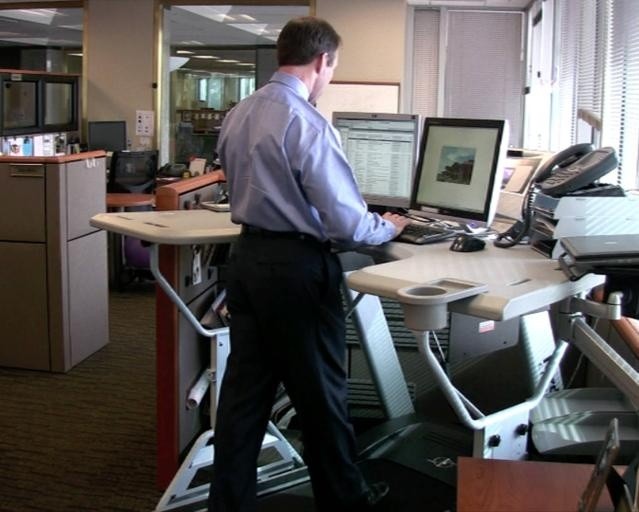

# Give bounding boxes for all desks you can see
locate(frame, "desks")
[93,191,599,512]
[104,191,157,267]
[454,455,639,511]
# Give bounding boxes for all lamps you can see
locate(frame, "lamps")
[168,57,190,75]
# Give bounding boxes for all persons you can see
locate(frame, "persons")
[206,18,412,510]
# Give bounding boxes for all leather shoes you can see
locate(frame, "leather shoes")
[368,482,389,504]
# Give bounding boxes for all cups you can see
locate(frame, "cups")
[183,111,220,121]
[72,143,80,153]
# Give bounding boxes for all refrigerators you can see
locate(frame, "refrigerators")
[1,150,110,374]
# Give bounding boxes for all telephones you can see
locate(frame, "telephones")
[162,164,187,177]
[533,144,618,194]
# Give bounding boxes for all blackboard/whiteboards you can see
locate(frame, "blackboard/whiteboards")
[314,81,400,126]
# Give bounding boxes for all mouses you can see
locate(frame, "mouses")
[450,234,485,251]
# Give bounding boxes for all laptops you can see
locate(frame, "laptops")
[560,233,639,267]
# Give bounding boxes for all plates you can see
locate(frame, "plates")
[201,201,230,212]
[213,125,221,130]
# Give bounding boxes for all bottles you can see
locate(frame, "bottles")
[182,158,222,179]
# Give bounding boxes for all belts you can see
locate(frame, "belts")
[240,225,318,245]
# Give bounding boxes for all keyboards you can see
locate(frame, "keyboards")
[392,224,455,244]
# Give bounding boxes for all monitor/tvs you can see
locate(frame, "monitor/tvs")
[42,77,78,133]
[409,117,510,234]
[87,121,127,151]
[0,74,42,136]
[332,111,422,209]
